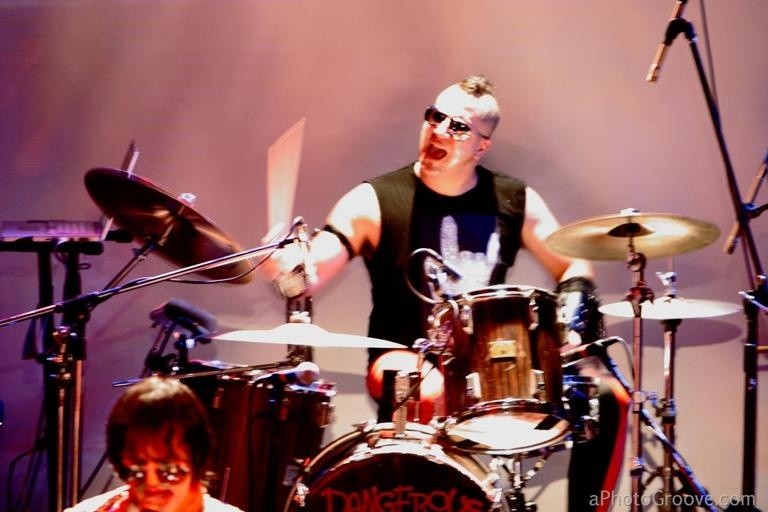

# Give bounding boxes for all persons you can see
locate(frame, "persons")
[293,76,595,368]
[62,377,242,512]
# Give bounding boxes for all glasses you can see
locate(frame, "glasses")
[422,104,491,142]
[120,463,192,487]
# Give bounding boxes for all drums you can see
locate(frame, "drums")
[181,360,337,511]
[286,423,515,511]
[426,286,577,456]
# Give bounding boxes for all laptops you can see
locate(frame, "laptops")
[0,140,140,244]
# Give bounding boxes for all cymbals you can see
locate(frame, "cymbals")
[547,213,721,261]
[210,323,409,350]
[598,296,742,319]
[83,167,256,284]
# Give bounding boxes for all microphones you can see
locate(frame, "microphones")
[299,297,314,361]
[426,247,463,285]
[297,222,318,288]
[560,336,619,364]
[646,0,689,84]
[254,362,321,387]
